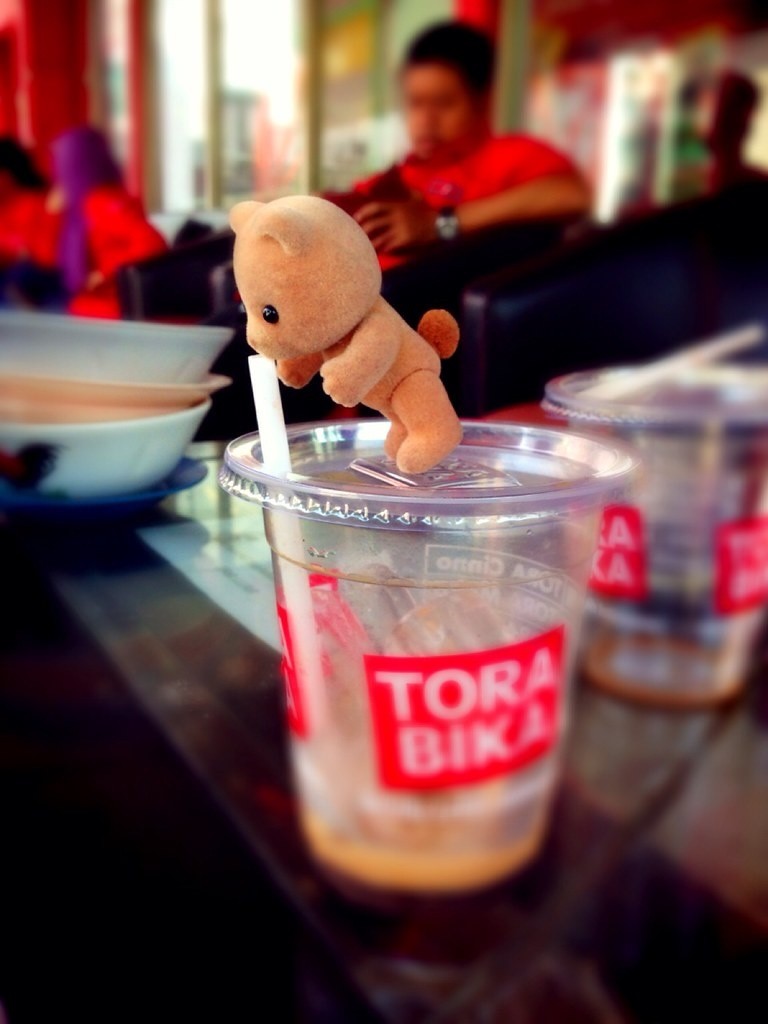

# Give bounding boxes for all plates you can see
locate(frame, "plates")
[0,457,208,506]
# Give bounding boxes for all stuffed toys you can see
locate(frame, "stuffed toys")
[228,194,465,475]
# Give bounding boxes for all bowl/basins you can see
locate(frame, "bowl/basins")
[0,313,233,491]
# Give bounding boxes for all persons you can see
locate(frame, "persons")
[609,66,768,224]
[0,124,171,322]
[319,18,592,271]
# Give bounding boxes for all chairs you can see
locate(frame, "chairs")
[115,174,768,442]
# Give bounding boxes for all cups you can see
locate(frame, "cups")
[223,419,643,889]
[544,367,768,702]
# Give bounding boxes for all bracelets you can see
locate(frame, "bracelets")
[438,204,458,238]
